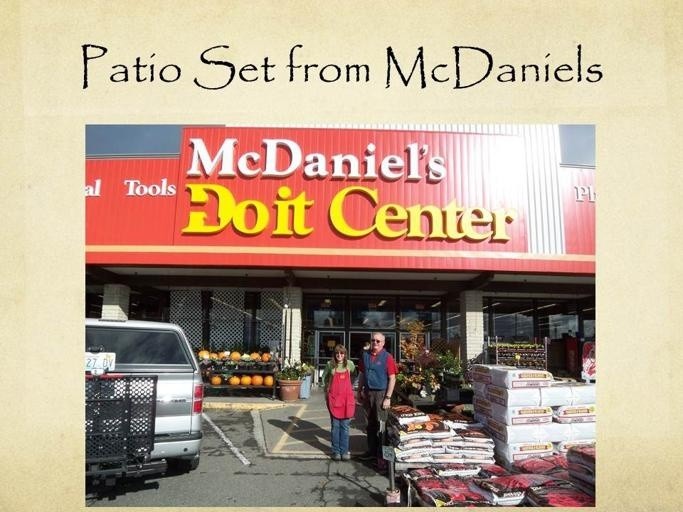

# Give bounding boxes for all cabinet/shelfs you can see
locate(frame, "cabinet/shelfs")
[485,335,548,370]
[201,360,278,399]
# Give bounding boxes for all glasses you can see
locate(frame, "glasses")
[372,339,380,343]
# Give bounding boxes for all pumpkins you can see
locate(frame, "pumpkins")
[198,350,274,385]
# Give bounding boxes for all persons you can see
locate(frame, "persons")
[356,332,398,460]
[359,342,370,358]
[320,345,357,461]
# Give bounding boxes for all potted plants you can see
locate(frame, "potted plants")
[275,363,314,401]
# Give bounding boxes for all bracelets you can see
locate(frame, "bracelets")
[385,395,391,400]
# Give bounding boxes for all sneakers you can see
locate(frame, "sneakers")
[357,452,376,460]
[332,454,351,460]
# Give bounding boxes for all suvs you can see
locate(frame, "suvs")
[85,314,206,479]
